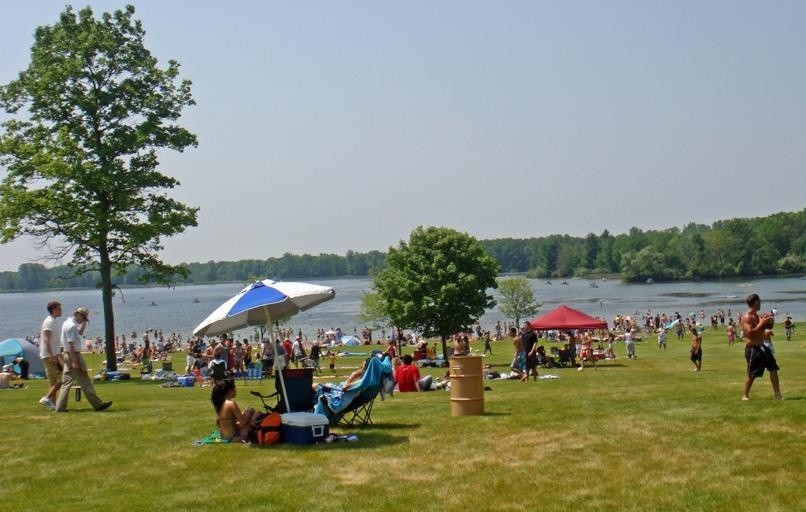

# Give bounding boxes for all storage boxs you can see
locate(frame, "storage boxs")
[106,371,121,381]
[177,376,195,387]
[276,411,332,445]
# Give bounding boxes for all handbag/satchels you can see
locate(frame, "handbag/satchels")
[258,412,281,447]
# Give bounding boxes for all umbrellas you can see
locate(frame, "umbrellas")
[193,278,336,412]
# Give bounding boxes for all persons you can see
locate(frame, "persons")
[0,332,41,389]
[742,294,783,401]
[39,301,62,409]
[211,377,265,443]
[55,307,112,411]
[763,308,795,340]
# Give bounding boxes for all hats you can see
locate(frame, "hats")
[74,307,88,323]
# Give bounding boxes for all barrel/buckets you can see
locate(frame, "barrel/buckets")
[448,355,484,417]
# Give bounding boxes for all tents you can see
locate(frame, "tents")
[0,338,59,379]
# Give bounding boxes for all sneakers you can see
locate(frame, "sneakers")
[39,396,55,409]
[94,400,112,410]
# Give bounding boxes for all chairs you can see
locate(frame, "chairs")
[558,349,571,370]
[533,352,548,369]
[318,357,390,430]
[233,368,262,386]
[250,367,317,413]
